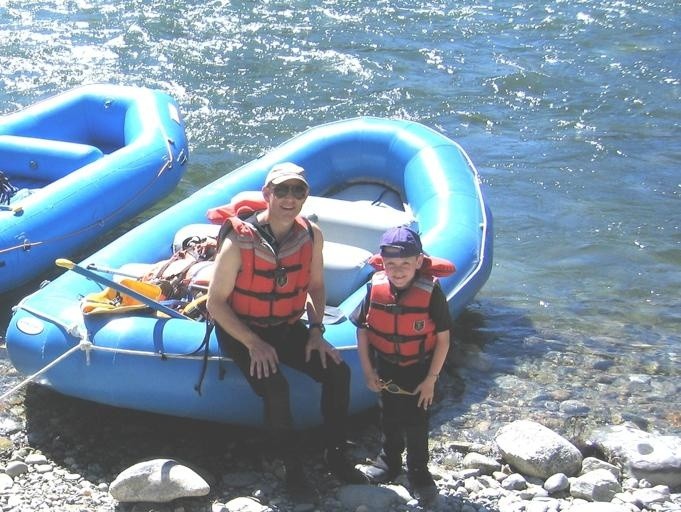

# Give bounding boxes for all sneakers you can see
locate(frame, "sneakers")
[282,460,321,504]
[407,469,437,501]
[367,448,402,483]
[325,441,370,485]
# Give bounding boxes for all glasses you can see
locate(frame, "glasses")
[378,377,416,397]
[273,183,306,199]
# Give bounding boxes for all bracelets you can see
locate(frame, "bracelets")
[429,372,441,380]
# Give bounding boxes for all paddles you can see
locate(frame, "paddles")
[87,261,345,324]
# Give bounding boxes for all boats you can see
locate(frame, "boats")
[0,83,190,286]
[4,111,496,431]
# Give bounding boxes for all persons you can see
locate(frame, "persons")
[349,225,450,502]
[207,161,370,506]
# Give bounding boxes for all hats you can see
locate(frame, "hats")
[263,162,310,196]
[379,225,422,259]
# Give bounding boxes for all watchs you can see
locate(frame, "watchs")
[307,322,327,332]
